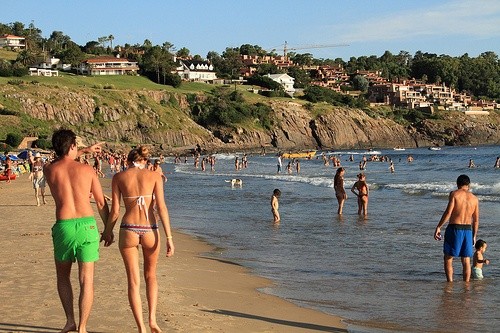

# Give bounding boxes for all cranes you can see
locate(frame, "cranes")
[269,41,352,57]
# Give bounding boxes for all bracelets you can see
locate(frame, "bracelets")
[166,236,173,239]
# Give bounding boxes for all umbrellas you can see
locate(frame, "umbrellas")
[2,155,18,160]
[17,151,34,160]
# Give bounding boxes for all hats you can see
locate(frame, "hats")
[35,153,41,158]
[28,151,34,154]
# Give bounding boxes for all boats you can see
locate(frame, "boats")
[281,149,316,158]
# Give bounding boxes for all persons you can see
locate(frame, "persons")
[286,148,312,175]
[0,150,57,184]
[391,163,395,173]
[261,147,265,157]
[496,157,500,168]
[42,130,115,333]
[100,146,175,333]
[224,178,243,188]
[322,148,353,168]
[31,152,47,205]
[79,144,168,211]
[334,167,348,215]
[271,188,280,222]
[351,172,369,215]
[359,161,363,170]
[407,155,413,164]
[432,174,479,282]
[471,239,489,281]
[363,154,393,163]
[235,154,248,171]
[469,159,475,168]
[363,161,367,170]
[276,154,282,174]
[175,143,216,172]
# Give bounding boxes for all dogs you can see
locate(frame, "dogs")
[224,178,243,189]
